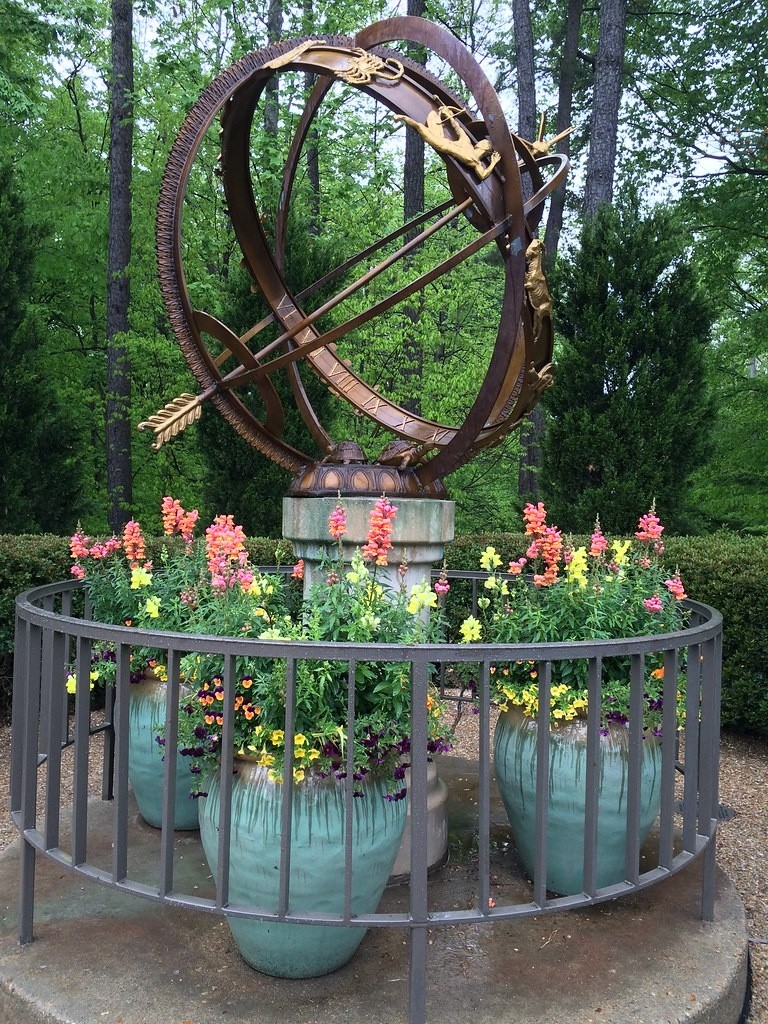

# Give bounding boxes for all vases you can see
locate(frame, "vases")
[113,668,214,830]
[493,695,662,896]
[196,743,407,980]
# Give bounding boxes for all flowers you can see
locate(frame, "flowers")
[62,491,692,801]
